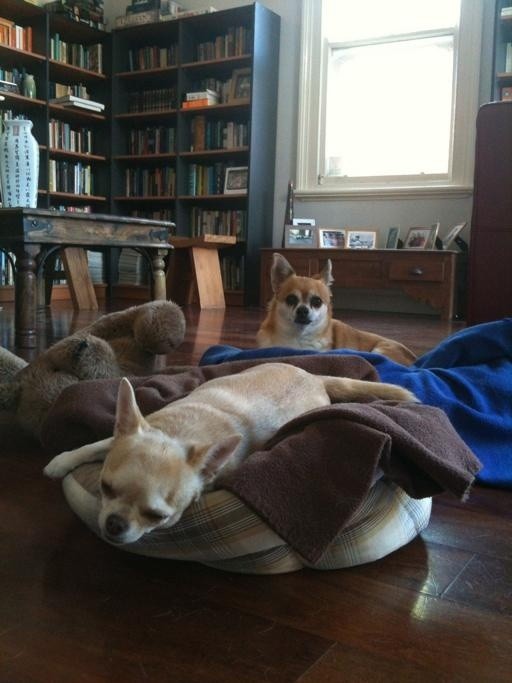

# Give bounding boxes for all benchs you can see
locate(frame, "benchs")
[169,233,237,309]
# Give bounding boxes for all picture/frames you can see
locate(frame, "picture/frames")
[384,226,404,250]
[292,217,316,226]
[431,221,471,251]
[318,228,347,248]
[403,226,434,249]
[346,229,377,250]
[284,225,319,248]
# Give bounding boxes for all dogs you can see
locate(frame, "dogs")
[256,252,419,367]
[44,365,419,548]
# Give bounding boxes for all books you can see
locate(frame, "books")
[197,23,255,60]
[220,249,248,291]
[126,124,176,157]
[125,206,177,234]
[1,12,38,54]
[124,88,180,112]
[128,39,181,72]
[46,197,93,216]
[181,74,234,108]
[0,111,31,137]
[51,77,105,112]
[51,29,104,71]
[117,247,143,288]
[188,206,245,239]
[182,162,226,195]
[122,166,175,195]
[0,253,19,288]
[85,249,107,288]
[187,117,249,153]
[1,58,38,99]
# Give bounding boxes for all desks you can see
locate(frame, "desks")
[0,207,177,349]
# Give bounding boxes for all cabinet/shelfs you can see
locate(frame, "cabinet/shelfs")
[478,0,512,108]
[0,0,282,310]
[258,248,464,321]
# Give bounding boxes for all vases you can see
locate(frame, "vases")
[0,120,40,208]
